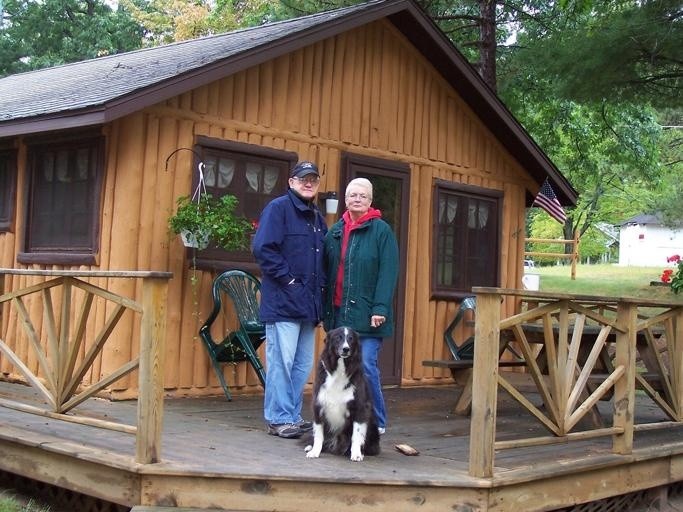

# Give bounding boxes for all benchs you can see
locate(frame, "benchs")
[574,371,670,428]
[420,358,529,415]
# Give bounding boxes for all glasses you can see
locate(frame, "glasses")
[293,177,318,184]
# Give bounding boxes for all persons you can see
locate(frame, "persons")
[324,178,399,435]
[251,162,329,438]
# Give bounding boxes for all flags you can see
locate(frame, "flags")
[534,180,568,225]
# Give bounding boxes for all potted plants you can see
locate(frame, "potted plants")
[167,191,259,374]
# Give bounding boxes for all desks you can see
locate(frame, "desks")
[449,324,671,429]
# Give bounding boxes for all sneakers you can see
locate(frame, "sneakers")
[267,416,314,439]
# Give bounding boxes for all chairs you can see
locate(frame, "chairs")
[443,297,527,369]
[198,269,273,401]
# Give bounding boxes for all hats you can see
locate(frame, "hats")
[287,160,320,178]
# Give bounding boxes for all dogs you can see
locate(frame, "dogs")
[297,323,381,463]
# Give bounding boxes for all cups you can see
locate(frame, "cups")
[522,274,539,291]
